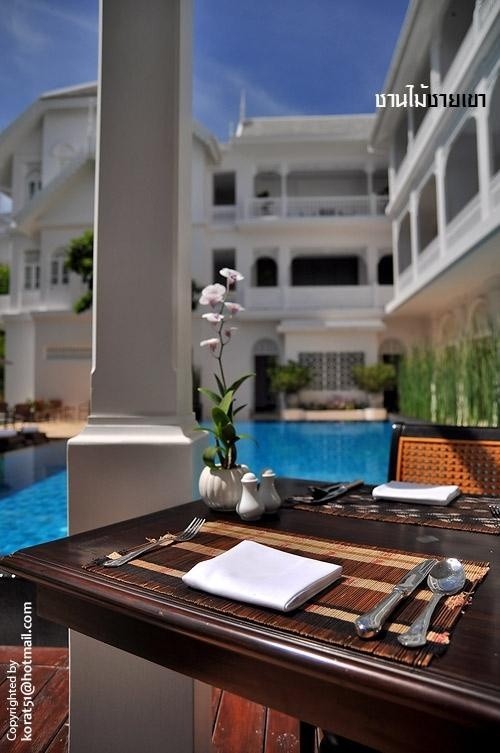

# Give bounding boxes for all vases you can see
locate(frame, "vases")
[194,458,254,515]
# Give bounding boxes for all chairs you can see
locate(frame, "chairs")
[308,411,500,753]
[0,397,91,427]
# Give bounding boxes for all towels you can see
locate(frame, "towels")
[370,474,465,509]
[176,537,349,617]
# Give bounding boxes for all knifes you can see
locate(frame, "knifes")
[291,478,365,505]
[352,557,437,638]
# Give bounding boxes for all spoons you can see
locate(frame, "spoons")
[395,556,465,648]
[307,483,345,495]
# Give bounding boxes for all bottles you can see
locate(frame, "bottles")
[235,471,265,523]
[258,468,283,514]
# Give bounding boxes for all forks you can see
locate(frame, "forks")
[101,516,205,569]
[489,504,500,518]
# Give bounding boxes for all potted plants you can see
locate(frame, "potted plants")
[266,355,316,409]
[346,360,400,410]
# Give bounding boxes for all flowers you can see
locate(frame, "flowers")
[188,258,256,470]
